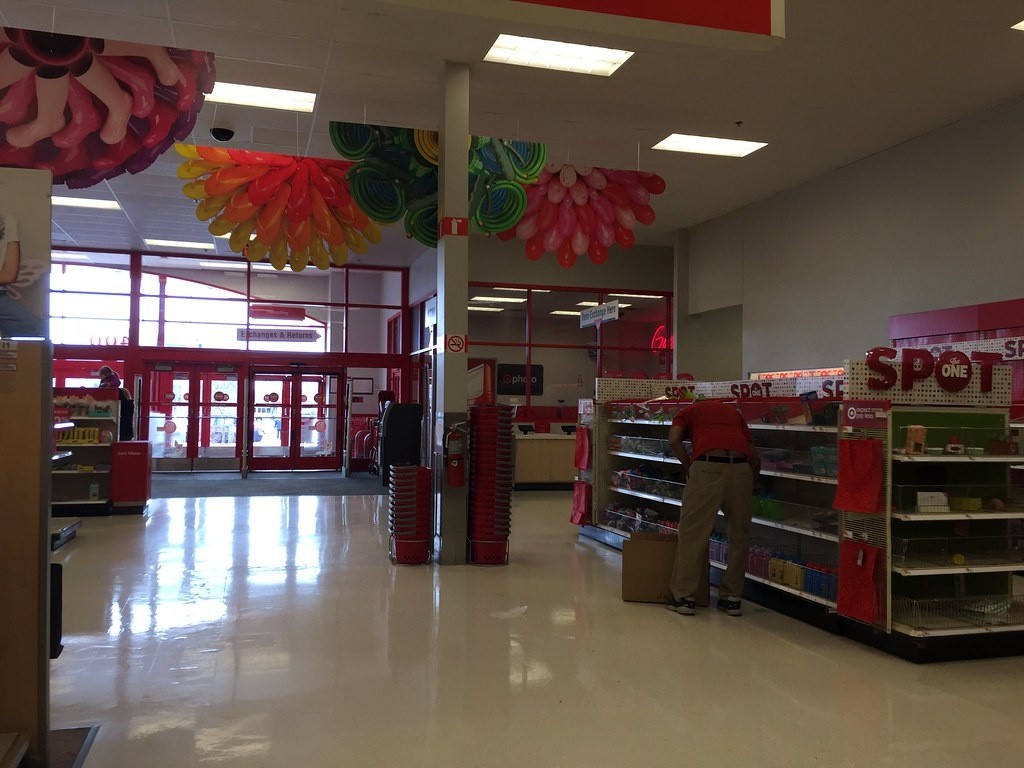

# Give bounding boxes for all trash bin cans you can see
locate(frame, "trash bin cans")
[378,402,423,488]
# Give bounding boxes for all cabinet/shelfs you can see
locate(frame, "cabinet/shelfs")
[568,347,1024,666]
[51,387,153,518]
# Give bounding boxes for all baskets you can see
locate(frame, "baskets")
[387,464,433,565]
[465,403,517,564]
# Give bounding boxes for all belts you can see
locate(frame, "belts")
[694,455,750,464]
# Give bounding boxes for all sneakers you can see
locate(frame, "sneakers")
[668,595,697,615]
[717,597,742,616]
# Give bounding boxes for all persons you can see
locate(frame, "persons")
[274,417,281,440]
[98,366,121,388]
[665,400,756,617]
[121,387,134,442]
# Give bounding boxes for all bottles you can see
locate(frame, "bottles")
[658,520,838,603]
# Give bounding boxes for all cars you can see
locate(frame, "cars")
[210,415,265,444]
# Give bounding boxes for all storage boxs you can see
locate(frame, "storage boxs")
[606,397,804,424]
[808,396,842,427]
[622,531,679,603]
[608,433,840,479]
[645,479,836,534]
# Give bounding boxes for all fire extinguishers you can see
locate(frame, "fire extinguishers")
[442,420,467,489]
